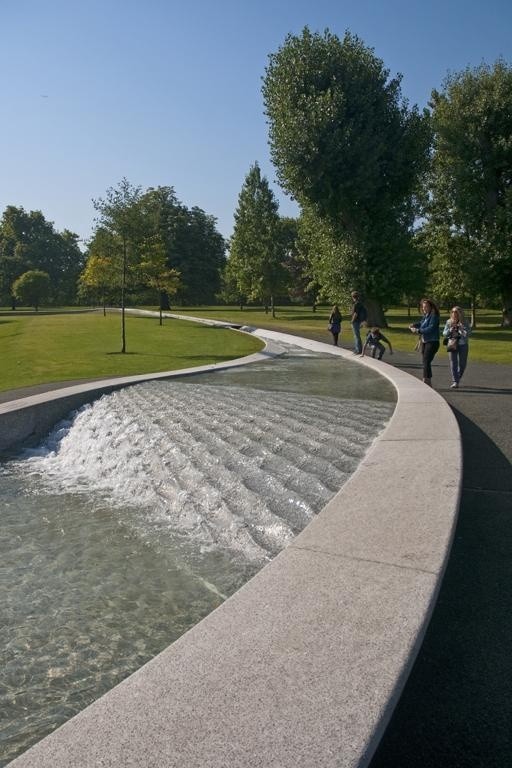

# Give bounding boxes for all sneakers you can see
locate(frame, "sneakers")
[449,382,458,388]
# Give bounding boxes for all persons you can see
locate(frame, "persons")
[329,290,394,360]
[407,298,470,388]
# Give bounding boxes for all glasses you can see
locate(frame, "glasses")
[451,311,457,313]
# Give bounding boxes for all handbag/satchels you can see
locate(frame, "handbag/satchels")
[414,340,423,353]
[447,337,457,352]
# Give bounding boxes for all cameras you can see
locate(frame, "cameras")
[454,326,459,332]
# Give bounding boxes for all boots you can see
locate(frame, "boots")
[423,377,432,388]
